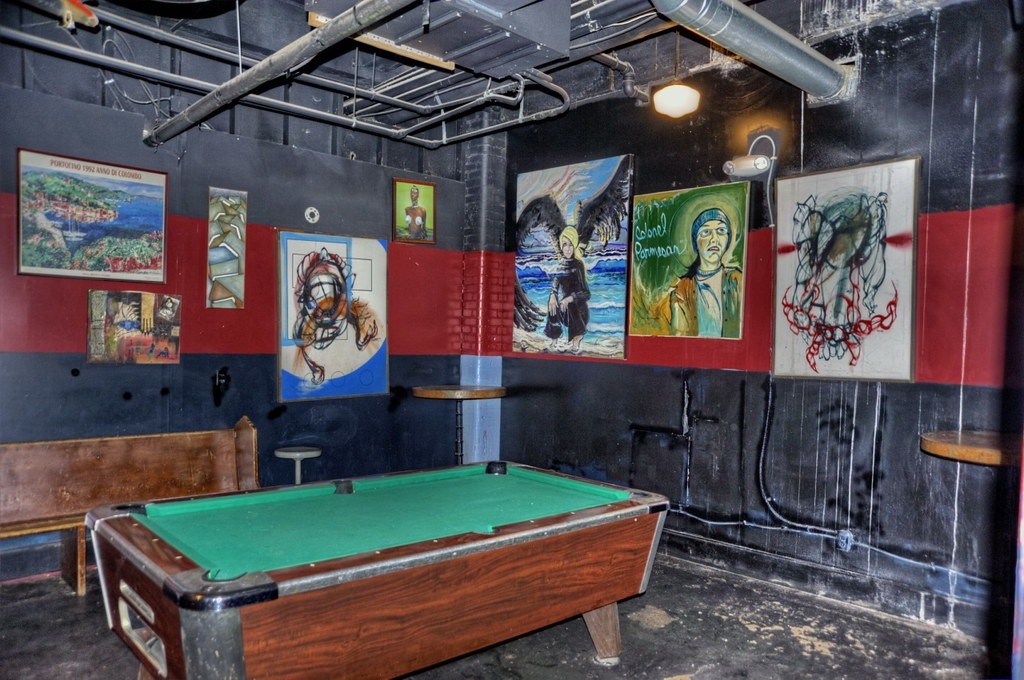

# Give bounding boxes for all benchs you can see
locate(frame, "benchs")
[0,416,259,595]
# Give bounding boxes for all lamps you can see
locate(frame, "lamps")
[653,29,701,118]
[722,154,773,179]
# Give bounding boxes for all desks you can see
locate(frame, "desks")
[83,460,670,680]
[413,385,507,464]
[920,432,1020,466]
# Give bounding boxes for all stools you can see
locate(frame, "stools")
[275,447,322,485]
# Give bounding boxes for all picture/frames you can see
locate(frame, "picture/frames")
[392,177,437,244]
[15,147,169,284]
